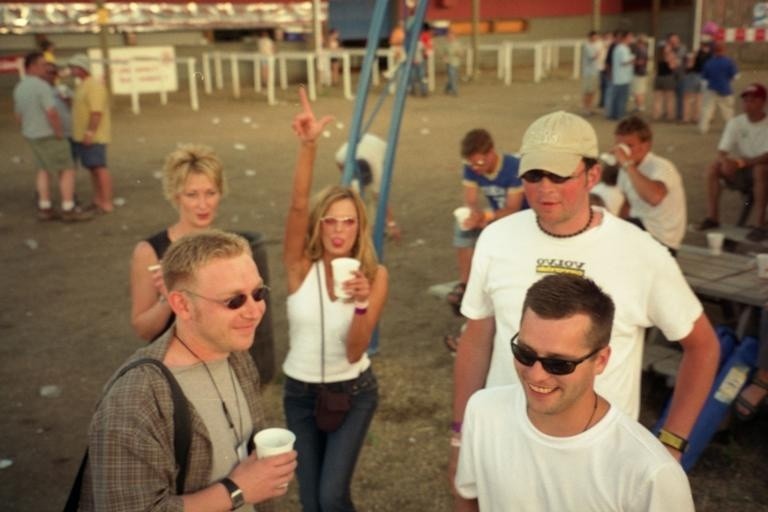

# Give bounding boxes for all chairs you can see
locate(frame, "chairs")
[652,325,756,469]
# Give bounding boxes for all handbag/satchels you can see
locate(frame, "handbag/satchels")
[314,385,352,431]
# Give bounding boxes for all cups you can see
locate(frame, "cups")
[330,257,359,298]
[757,253,768,278]
[253,428,296,488]
[606,144,631,165]
[706,234,724,256]
[453,207,470,232]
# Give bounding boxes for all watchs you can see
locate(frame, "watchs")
[220,476,245,510]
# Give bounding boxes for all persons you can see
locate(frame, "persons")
[446,129,529,304]
[447,110,720,464]
[282,87,390,512]
[442,31,461,97]
[257,30,274,87]
[12,51,84,223]
[391,18,404,58]
[326,28,339,87]
[63,229,297,512]
[40,61,72,140]
[409,43,429,96]
[732,308,768,421]
[694,83,768,242]
[66,54,115,216]
[131,143,275,390]
[421,23,434,48]
[454,274,695,512]
[582,30,741,136]
[602,115,687,256]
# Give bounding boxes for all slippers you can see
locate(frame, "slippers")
[84,201,99,210]
[87,204,114,216]
[443,334,460,353]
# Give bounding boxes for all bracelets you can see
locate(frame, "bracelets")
[657,428,688,453]
[484,209,495,222]
[354,300,370,315]
[623,159,635,171]
[85,128,96,137]
[450,436,462,448]
[736,157,747,169]
[451,421,462,433]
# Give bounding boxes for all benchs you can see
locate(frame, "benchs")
[653,352,683,377]
[688,220,768,247]
[428,280,677,375]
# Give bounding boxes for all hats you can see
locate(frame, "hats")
[740,83,767,99]
[516,108,599,178]
[714,42,725,53]
[68,54,91,74]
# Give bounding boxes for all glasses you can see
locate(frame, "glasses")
[510,331,607,376]
[460,151,487,167]
[317,217,357,228]
[521,166,587,186]
[45,69,57,75]
[170,285,271,310]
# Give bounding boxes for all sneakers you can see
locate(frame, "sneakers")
[35,206,62,220]
[746,222,768,243]
[59,204,96,224]
[693,218,721,231]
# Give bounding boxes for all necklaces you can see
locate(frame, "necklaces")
[537,208,594,237]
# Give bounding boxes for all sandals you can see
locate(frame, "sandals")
[446,282,466,306]
[732,369,766,420]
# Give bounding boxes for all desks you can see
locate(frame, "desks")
[673,244,759,287]
[700,266,768,308]
